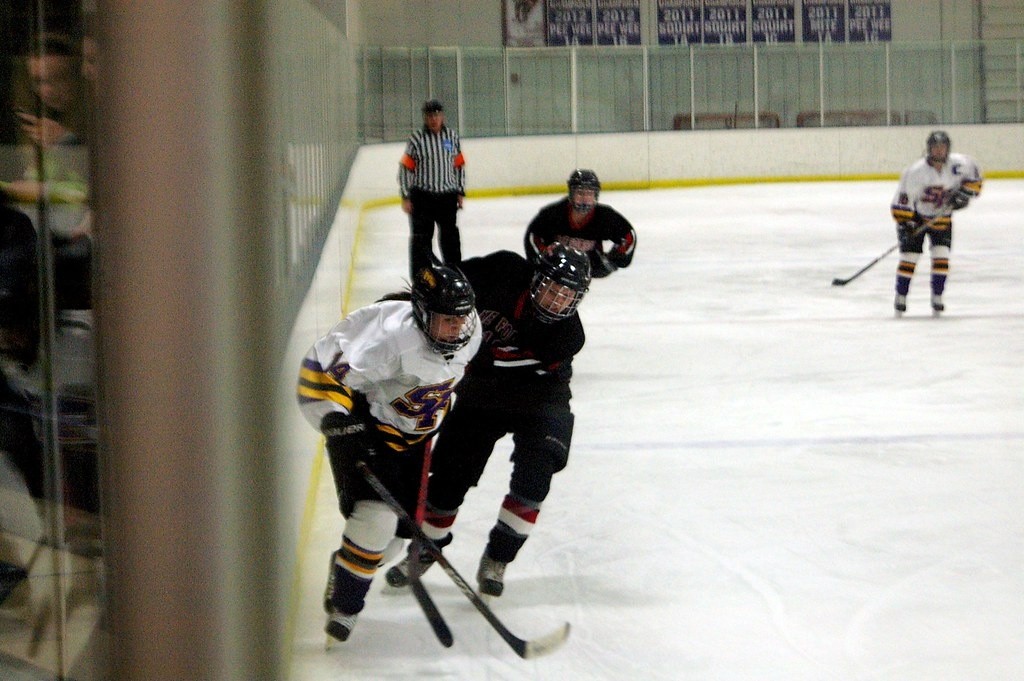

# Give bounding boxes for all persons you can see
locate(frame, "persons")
[397,99,466,284]
[0,32,105,609]
[297,265,483,639]
[385,240,591,597]
[524,170,637,278]
[891,130,982,311]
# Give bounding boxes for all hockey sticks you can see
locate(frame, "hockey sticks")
[406,438,457,648]
[358,460,571,661]
[832,203,953,287]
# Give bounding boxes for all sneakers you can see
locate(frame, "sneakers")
[476,547,507,606]
[893,292,906,316]
[382,543,442,596]
[323,551,362,650]
[931,291,944,316]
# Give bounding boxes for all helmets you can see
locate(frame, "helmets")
[567,169,602,213]
[412,266,478,355]
[926,131,950,166]
[526,241,592,325]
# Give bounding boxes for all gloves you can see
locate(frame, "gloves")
[898,222,915,245]
[322,412,376,471]
[591,255,618,278]
[949,188,976,209]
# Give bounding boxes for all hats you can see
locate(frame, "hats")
[421,100,442,113]
